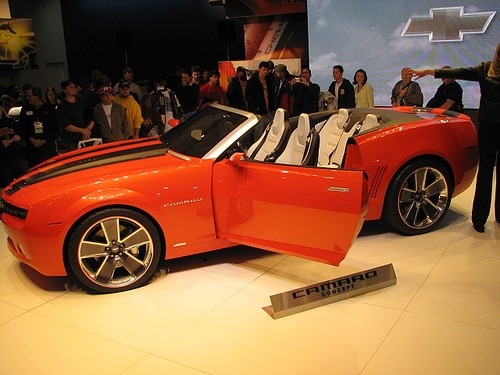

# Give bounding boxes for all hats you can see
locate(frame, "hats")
[119,81,130,88]
[61,81,73,89]
[98,87,112,95]
[237,66,248,72]
[123,68,131,74]
[274,64,286,72]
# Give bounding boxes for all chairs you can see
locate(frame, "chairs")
[245,107,380,169]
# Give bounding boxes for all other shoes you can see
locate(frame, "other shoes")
[474,223,484,234]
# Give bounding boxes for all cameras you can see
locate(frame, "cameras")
[294,78,300,82]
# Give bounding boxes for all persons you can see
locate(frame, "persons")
[434,66,464,113]
[391,67,423,106]
[0,60,375,190]
[406,43,500,232]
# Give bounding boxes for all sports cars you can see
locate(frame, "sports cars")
[0,101,480,293]
